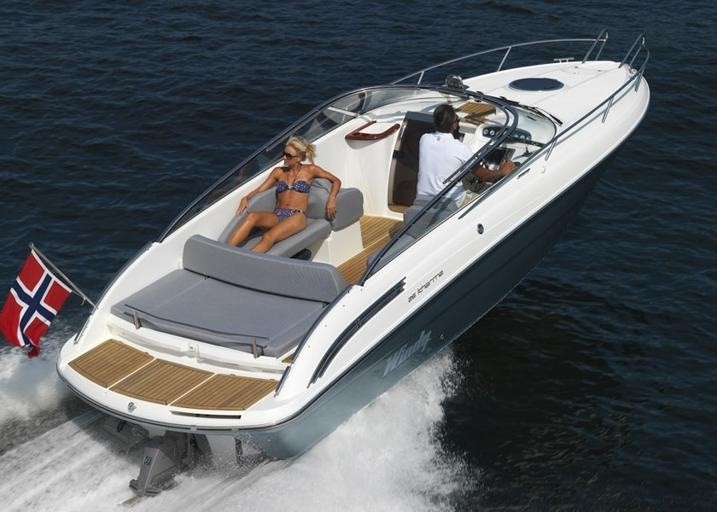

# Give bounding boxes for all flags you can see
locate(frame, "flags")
[0,250,75,360]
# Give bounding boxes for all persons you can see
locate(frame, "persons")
[225,137,341,257]
[414,103,518,213]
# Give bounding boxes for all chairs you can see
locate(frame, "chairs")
[366,191,457,273]
[218,181,364,262]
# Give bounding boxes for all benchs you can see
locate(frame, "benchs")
[110,234,348,358]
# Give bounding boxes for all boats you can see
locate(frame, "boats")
[29,27,651,492]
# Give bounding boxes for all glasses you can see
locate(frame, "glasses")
[452,115,458,123]
[282,151,297,160]
